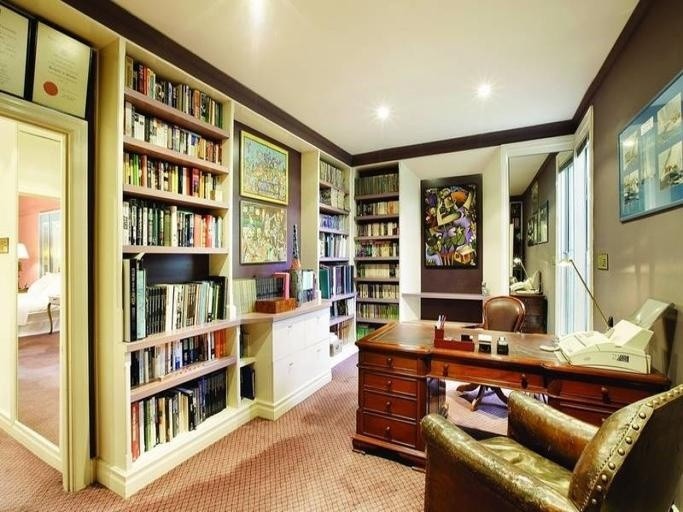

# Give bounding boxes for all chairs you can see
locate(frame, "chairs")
[417,380,682,512]
[454,297,527,412]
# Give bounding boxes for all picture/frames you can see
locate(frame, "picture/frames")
[526,178,550,248]
[614,69,683,224]
[422,182,479,270]
[239,128,290,207]
[237,199,287,267]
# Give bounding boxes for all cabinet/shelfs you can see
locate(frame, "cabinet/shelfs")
[509,200,524,282]
[351,190,400,326]
[90,42,252,503]
[240,301,334,423]
[299,148,358,367]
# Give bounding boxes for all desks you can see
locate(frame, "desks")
[348,319,673,474]
[44,296,62,336]
[510,269,547,333]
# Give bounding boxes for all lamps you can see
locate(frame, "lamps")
[512,257,535,292]
[15,242,31,293]
[558,251,611,326]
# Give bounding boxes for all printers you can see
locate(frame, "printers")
[558,319,655,375]
[510,270,541,292]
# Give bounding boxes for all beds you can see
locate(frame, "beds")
[17,269,63,338]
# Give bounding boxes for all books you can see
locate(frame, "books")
[124,201,222,249]
[319,159,400,355]
[126,153,222,201]
[125,56,222,130]
[130,328,227,389]
[125,101,221,165]
[123,259,227,342]
[131,369,228,462]
[231,269,316,315]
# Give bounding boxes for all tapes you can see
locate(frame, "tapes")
[500,336,504,341]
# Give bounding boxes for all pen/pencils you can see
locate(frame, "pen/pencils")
[436,314,446,329]
